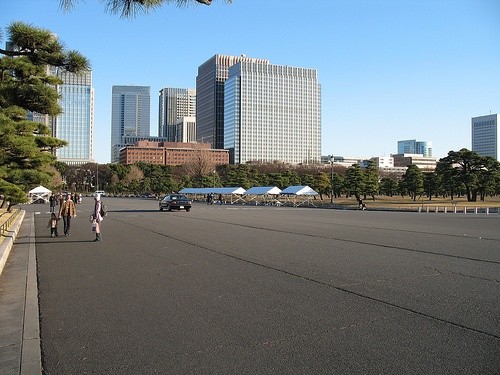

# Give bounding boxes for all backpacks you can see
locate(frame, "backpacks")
[95,201,108,217]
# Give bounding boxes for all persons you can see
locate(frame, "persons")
[92,194,103,242]
[219,194,222,206]
[359,198,366,210]
[47,213,61,237]
[49,193,58,213]
[58,194,76,236]
[58,192,82,206]
[207,192,214,205]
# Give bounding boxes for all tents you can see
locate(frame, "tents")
[243,185,323,207]
[28,185,52,204]
[178,187,247,204]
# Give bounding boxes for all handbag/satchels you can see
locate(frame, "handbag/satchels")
[59,204,65,215]
[92,219,96,231]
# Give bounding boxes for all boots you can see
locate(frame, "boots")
[94,233,101,242]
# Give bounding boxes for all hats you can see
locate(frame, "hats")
[95,193,100,201]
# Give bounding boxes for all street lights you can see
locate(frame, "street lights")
[329,156,334,200]
[213,170,216,188]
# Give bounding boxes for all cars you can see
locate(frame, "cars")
[114,192,168,201]
[158,193,193,212]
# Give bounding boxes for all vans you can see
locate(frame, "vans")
[93,190,106,197]
